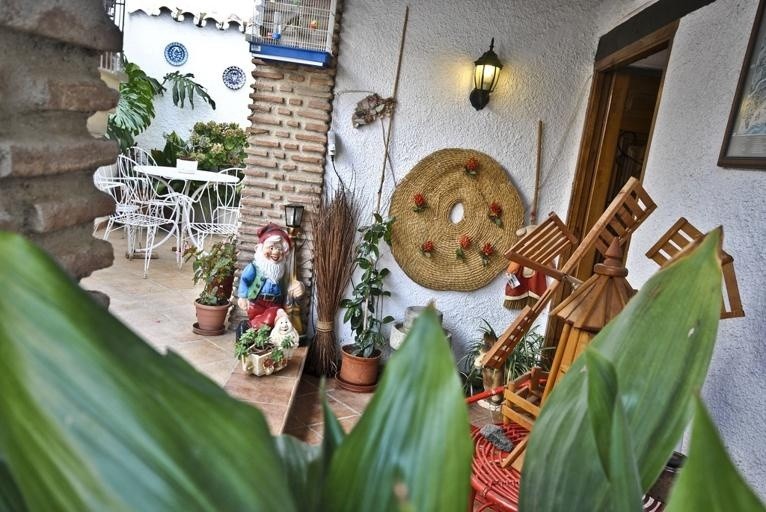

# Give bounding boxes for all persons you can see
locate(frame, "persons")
[236,222,308,343]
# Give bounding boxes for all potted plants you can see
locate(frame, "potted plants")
[334,213,393,393]
[177,235,237,336]
[235,326,281,377]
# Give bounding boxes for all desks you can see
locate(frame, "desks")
[467,421,534,512]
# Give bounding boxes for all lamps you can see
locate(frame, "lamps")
[469,37,502,110]
[278,202,306,346]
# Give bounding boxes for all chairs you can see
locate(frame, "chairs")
[92,145,246,280]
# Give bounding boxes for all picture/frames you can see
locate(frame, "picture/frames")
[717,1,764,168]
